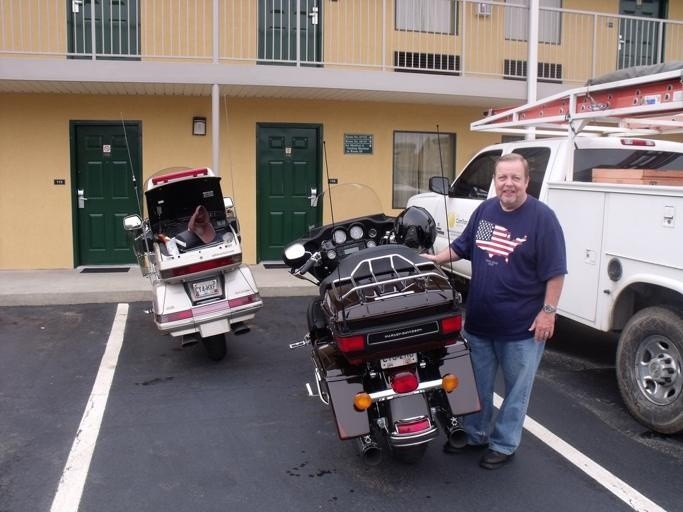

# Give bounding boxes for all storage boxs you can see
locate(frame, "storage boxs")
[592,168,683,185]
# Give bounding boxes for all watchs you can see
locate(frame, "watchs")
[542,304,556,313]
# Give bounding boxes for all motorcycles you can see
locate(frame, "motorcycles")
[122,165,264,360]
[283,183,483,467]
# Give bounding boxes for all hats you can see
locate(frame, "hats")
[187,204,217,245]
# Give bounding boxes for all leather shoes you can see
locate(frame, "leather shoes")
[442,440,489,456]
[478,449,516,470]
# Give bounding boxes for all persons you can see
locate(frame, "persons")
[417,151,568,473]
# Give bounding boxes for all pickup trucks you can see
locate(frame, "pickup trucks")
[407,137,683,435]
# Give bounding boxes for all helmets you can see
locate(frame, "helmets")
[397,207,437,250]
